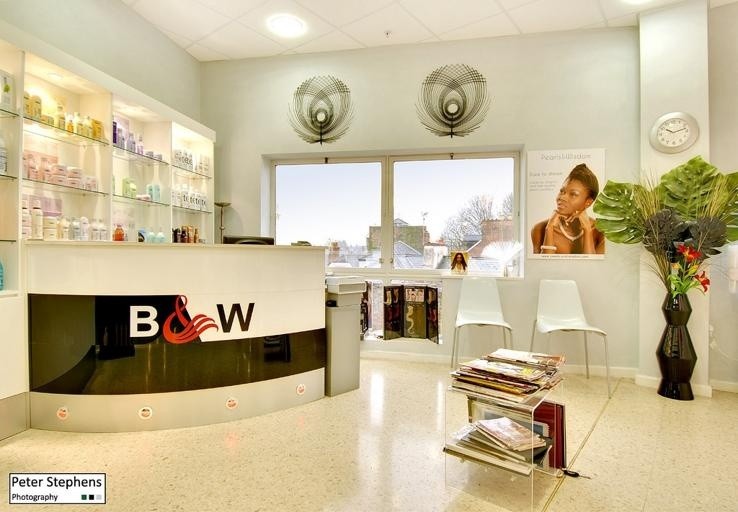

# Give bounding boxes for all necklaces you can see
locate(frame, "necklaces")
[558,222,584,241]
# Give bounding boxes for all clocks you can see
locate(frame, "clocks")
[649,112,701,155]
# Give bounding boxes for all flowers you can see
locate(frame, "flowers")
[593,156,738,296]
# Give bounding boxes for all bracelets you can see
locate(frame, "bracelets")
[541,246,557,251]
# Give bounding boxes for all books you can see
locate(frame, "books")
[446,347,567,474]
[359,278,444,348]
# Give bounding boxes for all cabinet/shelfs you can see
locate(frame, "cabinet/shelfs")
[445,379,568,512]
[0,39,215,242]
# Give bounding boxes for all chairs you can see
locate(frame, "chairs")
[449,276,613,401]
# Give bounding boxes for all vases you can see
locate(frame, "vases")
[655,292,698,401]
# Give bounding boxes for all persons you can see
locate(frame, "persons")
[451,253,467,273]
[531,163,605,254]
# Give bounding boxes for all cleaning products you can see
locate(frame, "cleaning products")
[22,199,108,239]
[24,91,107,142]
[172,183,208,212]
